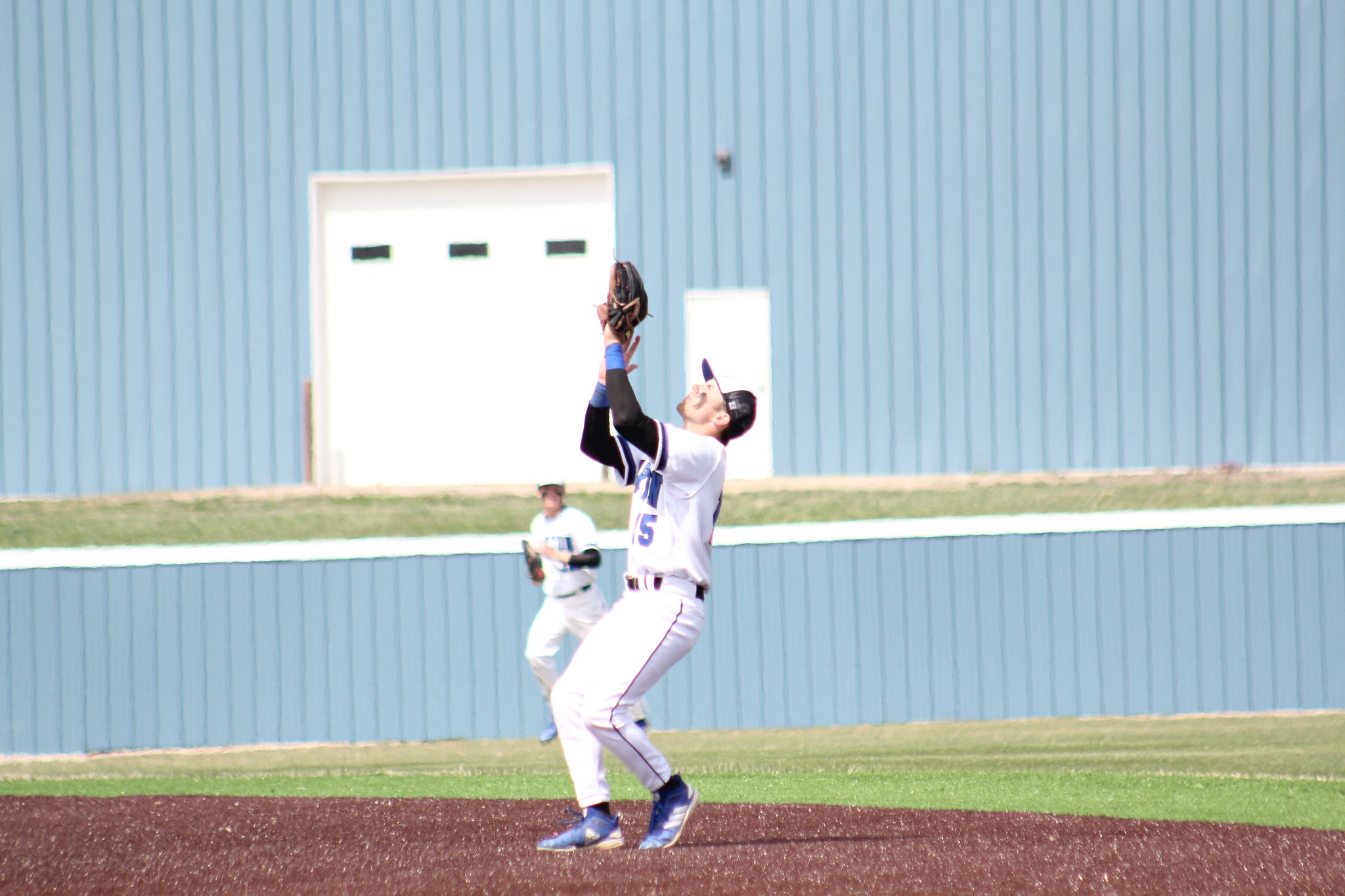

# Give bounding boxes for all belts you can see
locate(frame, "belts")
[557,585,591,598]
[628,577,705,599]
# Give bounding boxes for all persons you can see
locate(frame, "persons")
[537,303,756,851]
[523,478,647,743]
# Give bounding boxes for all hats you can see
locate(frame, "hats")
[702,358,757,439]
[538,474,566,490]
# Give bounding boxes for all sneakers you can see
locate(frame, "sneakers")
[540,722,558,742]
[638,770,699,849]
[536,807,624,851]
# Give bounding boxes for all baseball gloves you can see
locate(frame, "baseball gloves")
[520,539,545,583]
[592,247,656,345]
